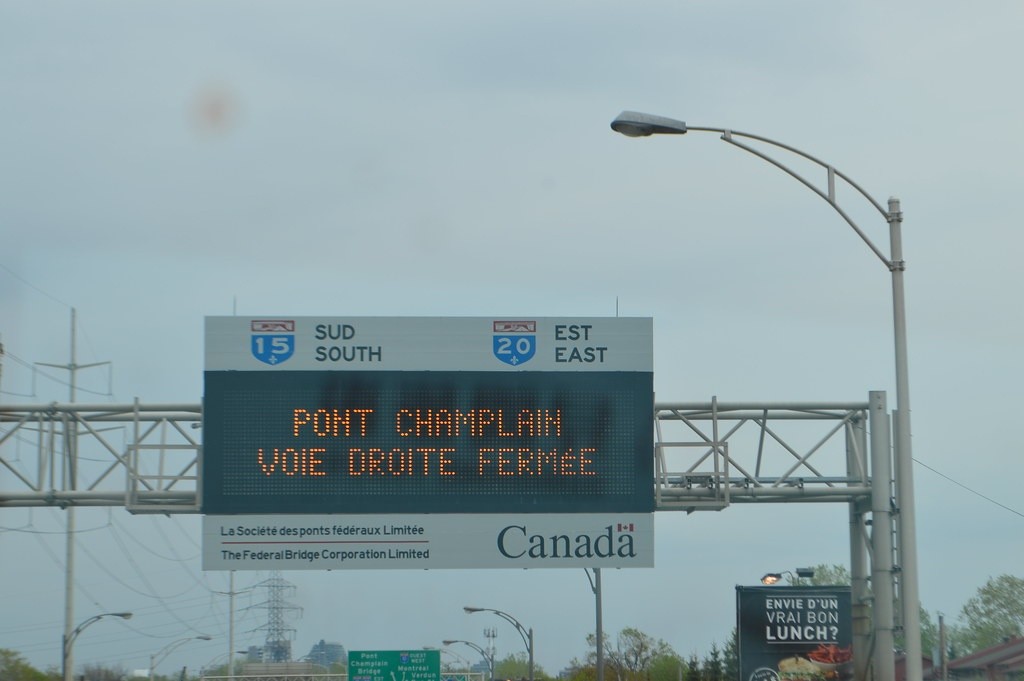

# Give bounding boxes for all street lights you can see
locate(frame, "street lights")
[442,639,497,681]
[149,636,213,681]
[609,108,924,681]
[199,650,248,681]
[424,646,471,681]
[61,612,134,681]
[463,607,534,681]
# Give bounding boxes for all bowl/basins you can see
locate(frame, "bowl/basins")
[809,659,853,676]
